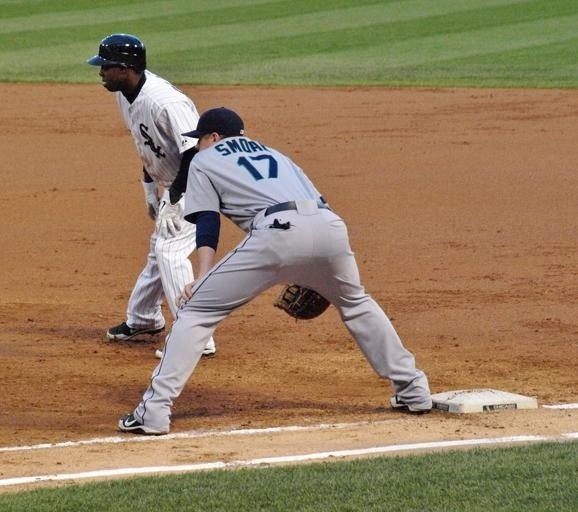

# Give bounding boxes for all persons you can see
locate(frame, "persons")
[85,32,216,358]
[116,106,433,436]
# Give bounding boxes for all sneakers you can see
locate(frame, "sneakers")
[390,394,433,414]
[105,320,165,342]
[117,413,171,437]
[154,334,217,360]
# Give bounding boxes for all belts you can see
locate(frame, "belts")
[264,201,330,218]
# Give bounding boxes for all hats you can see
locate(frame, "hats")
[180,106,245,138]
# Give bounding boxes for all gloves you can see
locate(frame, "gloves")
[142,182,159,222]
[156,187,184,239]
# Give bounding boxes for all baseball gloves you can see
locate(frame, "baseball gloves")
[273,282,331,322]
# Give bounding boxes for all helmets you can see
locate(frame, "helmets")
[85,34,147,73]
